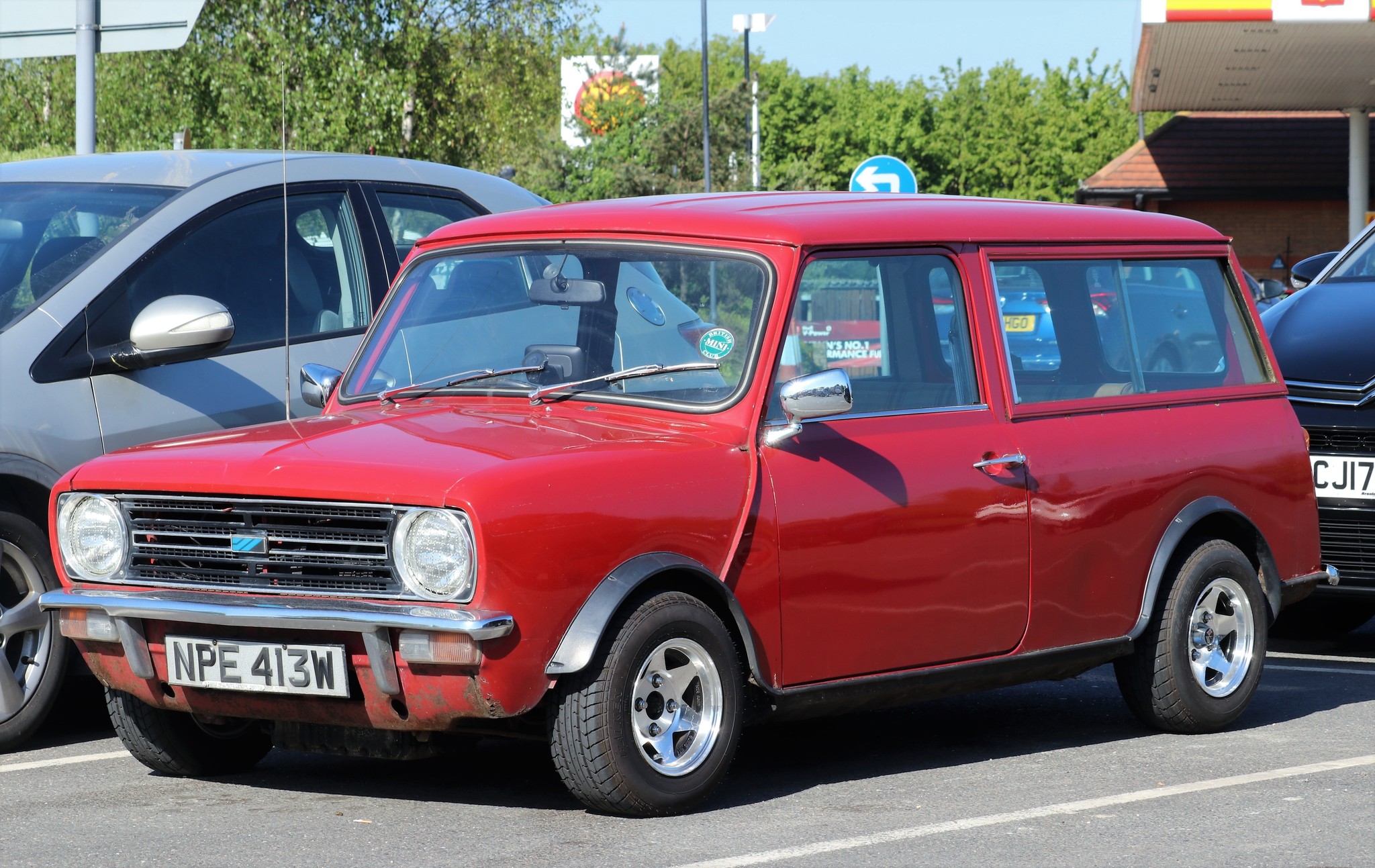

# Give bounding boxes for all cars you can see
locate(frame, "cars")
[3,144,737,734]
[923,255,1286,377]
[31,181,1334,816]
[1250,217,1375,603]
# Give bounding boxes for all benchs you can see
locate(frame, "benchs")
[851,378,1134,415]
[269,228,529,323]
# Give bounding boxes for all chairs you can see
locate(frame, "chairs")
[30,235,127,353]
